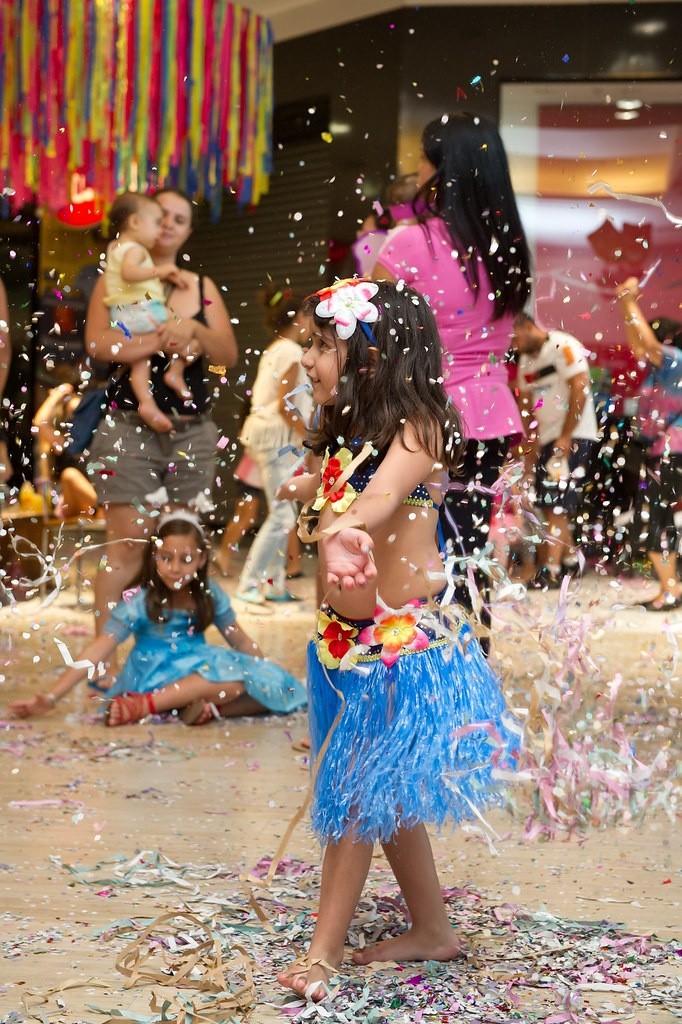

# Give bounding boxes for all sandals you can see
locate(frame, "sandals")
[103,691,155,727]
[177,699,212,726]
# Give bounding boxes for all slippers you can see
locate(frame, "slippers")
[89,676,114,694]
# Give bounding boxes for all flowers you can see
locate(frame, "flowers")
[315,281,380,339]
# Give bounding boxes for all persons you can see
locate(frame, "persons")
[618,275,682,614]
[272,278,531,1002]
[10,510,312,728]
[511,315,603,594]
[213,446,307,582]
[105,193,201,437]
[234,287,321,610]
[85,191,240,698]
[374,113,533,656]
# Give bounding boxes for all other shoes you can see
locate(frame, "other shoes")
[230,589,276,617]
[559,562,582,579]
[264,592,307,607]
[632,593,682,611]
[524,567,563,589]
